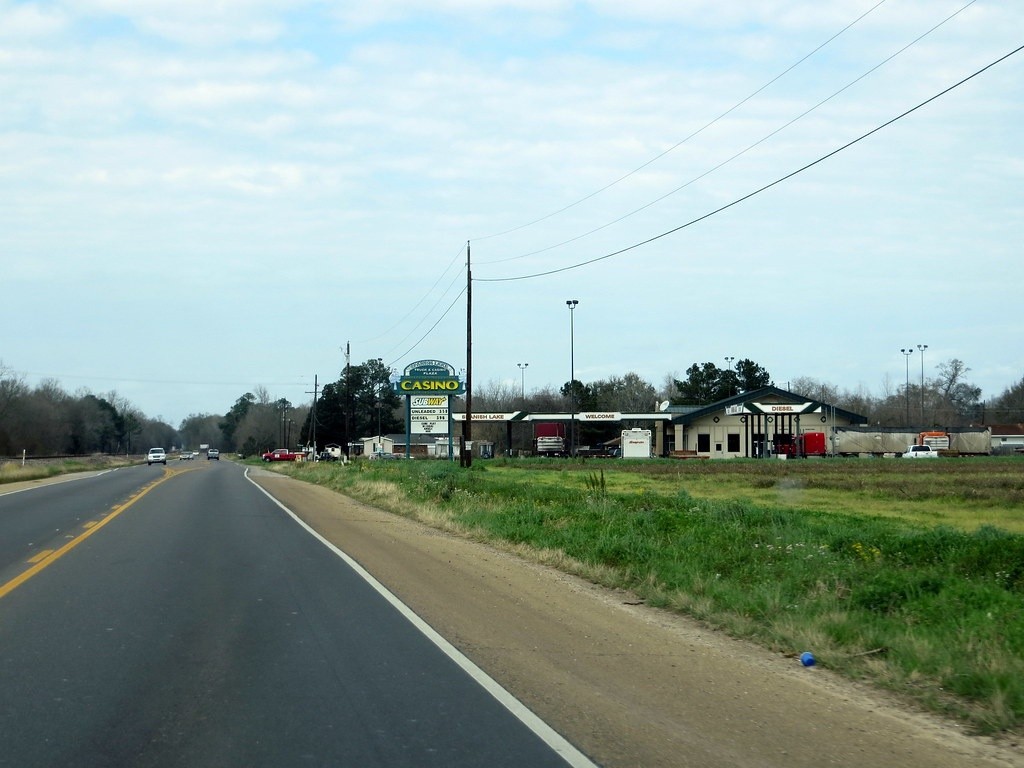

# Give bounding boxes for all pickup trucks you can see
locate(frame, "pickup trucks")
[263,449,305,463]
[902,445,937,458]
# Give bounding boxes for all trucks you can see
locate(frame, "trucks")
[791,432,826,458]
[532,422,566,457]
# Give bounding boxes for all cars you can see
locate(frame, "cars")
[180,452,190,460]
[579,447,621,457]
[146,447,167,465]
[193,449,199,456]
[207,449,219,460]
[188,452,194,460]
[316,452,338,463]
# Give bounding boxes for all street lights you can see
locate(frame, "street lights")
[917,345,928,426]
[517,363,528,459]
[725,356,734,397]
[566,300,578,458]
[901,349,913,427]
